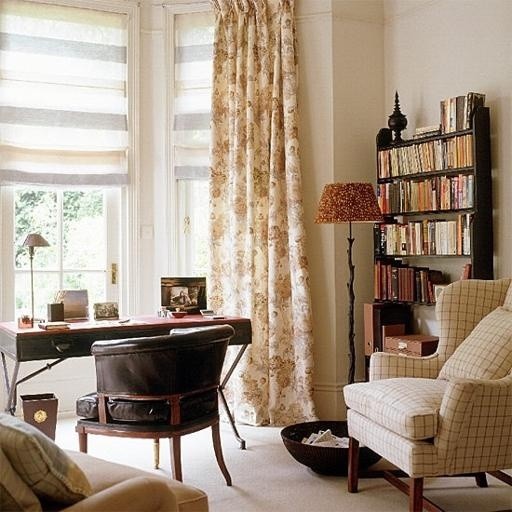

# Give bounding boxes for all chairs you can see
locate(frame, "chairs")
[72,325,234,488]
[0,452,209,512]
[344,279,511,511]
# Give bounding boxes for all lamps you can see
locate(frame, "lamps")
[23,233,50,317]
[318,182,387,384]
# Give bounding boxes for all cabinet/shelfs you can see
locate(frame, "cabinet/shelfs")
[374,104,492,302]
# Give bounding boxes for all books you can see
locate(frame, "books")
[375,92,486,304]
[382,324,405,352]
[38,321,70,330]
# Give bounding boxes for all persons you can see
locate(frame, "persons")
[172,291,191,305]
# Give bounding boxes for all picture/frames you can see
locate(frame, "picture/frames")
[161,277,206,313]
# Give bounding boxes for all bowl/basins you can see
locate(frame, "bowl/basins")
[171,312,188,319]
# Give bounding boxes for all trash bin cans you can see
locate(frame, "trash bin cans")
[19,392,58,440]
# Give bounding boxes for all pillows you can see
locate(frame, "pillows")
[0,414,93,502]
[436,306,512,385]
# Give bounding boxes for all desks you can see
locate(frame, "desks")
[0,314,252,451]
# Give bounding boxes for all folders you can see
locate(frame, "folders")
[363,302,411,357]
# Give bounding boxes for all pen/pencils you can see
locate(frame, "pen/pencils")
[212,317,225,319]
[120,319,130,323]
[54,290,69,303]
